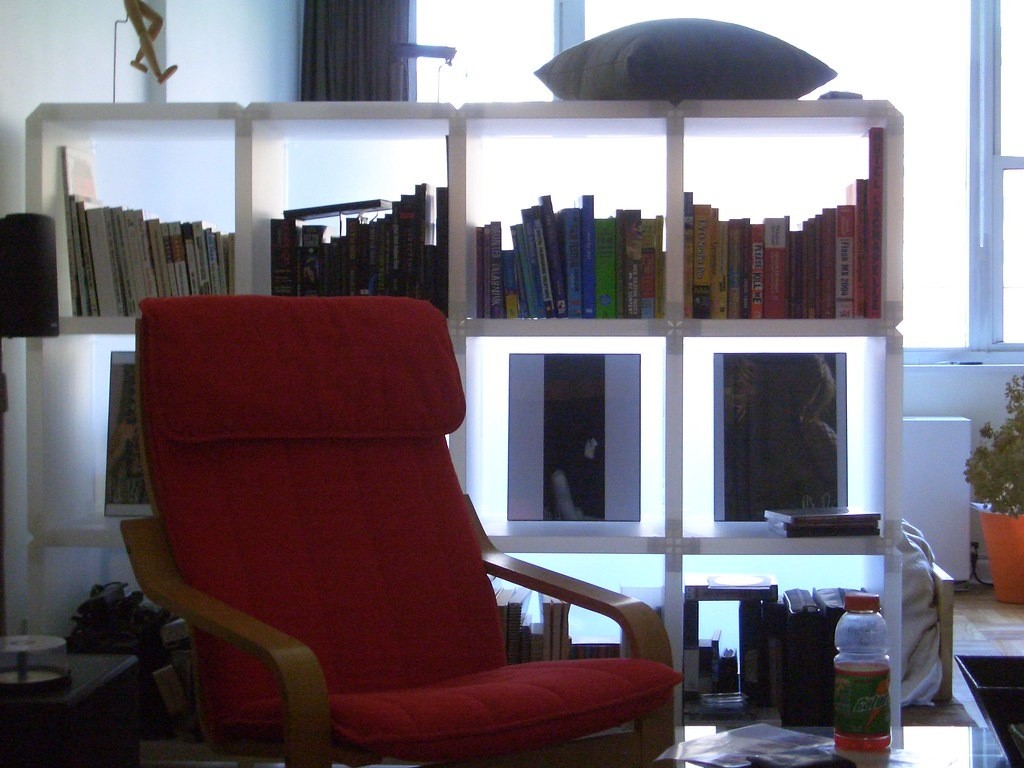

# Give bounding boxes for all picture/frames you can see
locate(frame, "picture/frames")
[103,350,154,517]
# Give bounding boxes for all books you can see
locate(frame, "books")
[62,145,234,317]
[490,576,620,665]
[475,196,667,319]
[764,509,881,536]
[270,183,449,318]
[684,177,881,367]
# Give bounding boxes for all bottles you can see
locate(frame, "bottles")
[834,593,891,751]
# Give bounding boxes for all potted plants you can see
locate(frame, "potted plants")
[963,374,1024,602]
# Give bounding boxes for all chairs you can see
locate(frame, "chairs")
[121,296,687,768]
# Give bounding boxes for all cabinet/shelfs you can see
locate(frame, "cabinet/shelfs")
[24,99,906,754]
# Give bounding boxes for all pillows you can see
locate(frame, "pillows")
[534,18,838,99]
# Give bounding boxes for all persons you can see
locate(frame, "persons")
[727,354,837,512]
[543,434,605,520]
